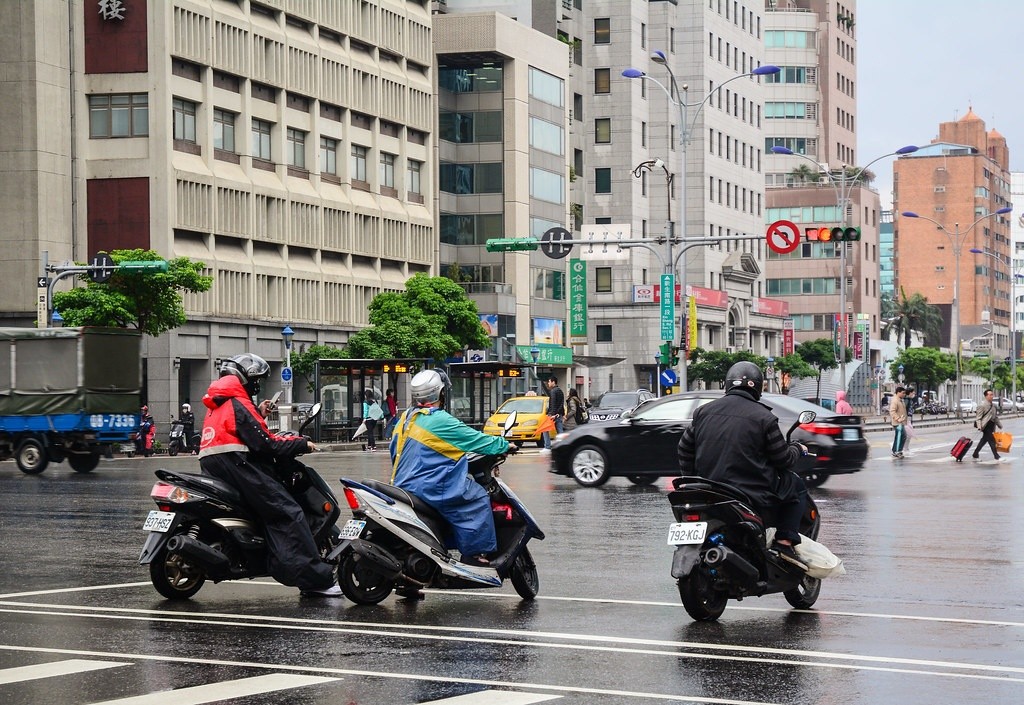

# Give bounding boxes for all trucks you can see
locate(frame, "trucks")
[0,324,142,474]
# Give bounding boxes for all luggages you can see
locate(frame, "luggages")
[950,428,982,462]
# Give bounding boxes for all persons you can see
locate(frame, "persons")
[388,367,517,567]
[197,353,344,598]
[179,403,197,455]
[677,361,809,572]
[135,404,157,457]
[972,389,1006,462]
[538,375,592,453]
[835,390,853,414]
[881,387,928,459]
[362,389,400,450]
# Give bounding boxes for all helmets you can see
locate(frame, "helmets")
[141,406,148,410]
[725,361,764,401]
[409,368,452,405]
[182,404,191,412]
[220,352,271,396]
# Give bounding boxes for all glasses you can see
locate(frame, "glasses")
[911,392,916,395]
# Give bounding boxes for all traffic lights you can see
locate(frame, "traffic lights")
[658,343,669,364]
[806,226,861,241]
[672,346,679,366]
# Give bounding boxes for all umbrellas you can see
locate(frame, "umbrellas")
[531,411,560,437]
[352,420,368,440]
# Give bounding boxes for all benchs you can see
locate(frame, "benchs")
[467,422,483,429]
[324,427,358,443]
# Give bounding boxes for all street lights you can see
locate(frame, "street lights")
[654,353,662,398]
[281,325,294,405]
[901,206,1013,417]
[898,363,905,384]
[970,248,1024,414]
[620,50,782,394]
[769,145,920,394]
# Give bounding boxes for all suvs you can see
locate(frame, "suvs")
[587,388,656,421]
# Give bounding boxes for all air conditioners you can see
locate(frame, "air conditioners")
[818,163,828,173]
[819,177,829,185]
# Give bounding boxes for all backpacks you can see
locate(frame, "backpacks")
[572,398,590,425]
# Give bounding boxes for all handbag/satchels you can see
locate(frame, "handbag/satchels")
[381,401,390,415]
[994,429,1013,453]
[973,421,977,427]
[368,402,384,420]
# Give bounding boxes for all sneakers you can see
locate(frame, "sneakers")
[768,540,809,571]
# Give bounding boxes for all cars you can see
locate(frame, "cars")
[548,389,869,488]
[483,391,564,448]
[989,397,1024,412]
[266,402,314,429]
[954,398,977,413]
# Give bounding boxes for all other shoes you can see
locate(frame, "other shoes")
[896,452,905,459]
[461,553,491,567]
[371,448,376,452]
[365,446,372,451]
[892,452,898,457]
[540,448,552,454]
[191,452,197,455]
[315,586,343,595]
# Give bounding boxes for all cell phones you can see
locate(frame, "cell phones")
[266,392,282,409]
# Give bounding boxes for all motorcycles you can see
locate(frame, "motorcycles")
[168,415,202,456]
[324,409,545,607]
[916,397,948,414]
[666,410,822,621]
[133,416,152,455]
[138,404,345,600]
